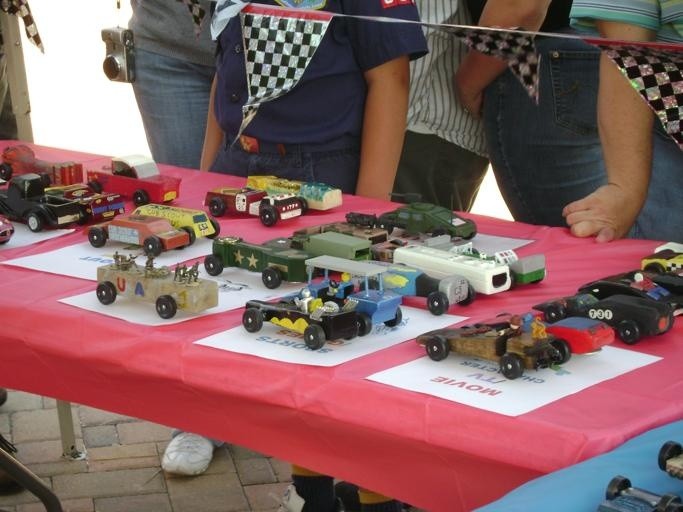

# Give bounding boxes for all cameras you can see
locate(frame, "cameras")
[101,26,137,82]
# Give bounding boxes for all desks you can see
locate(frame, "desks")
[0,139,683,512]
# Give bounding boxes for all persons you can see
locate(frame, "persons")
[124,0,236,477]
[1,0,42,408]
[392,1,491,211]
[199,2,431,511]
[563,1,682,242]
[456,1,683,243]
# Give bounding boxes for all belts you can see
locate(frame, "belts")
[226,132,361,154]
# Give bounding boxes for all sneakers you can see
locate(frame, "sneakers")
[162,432,214,477]
